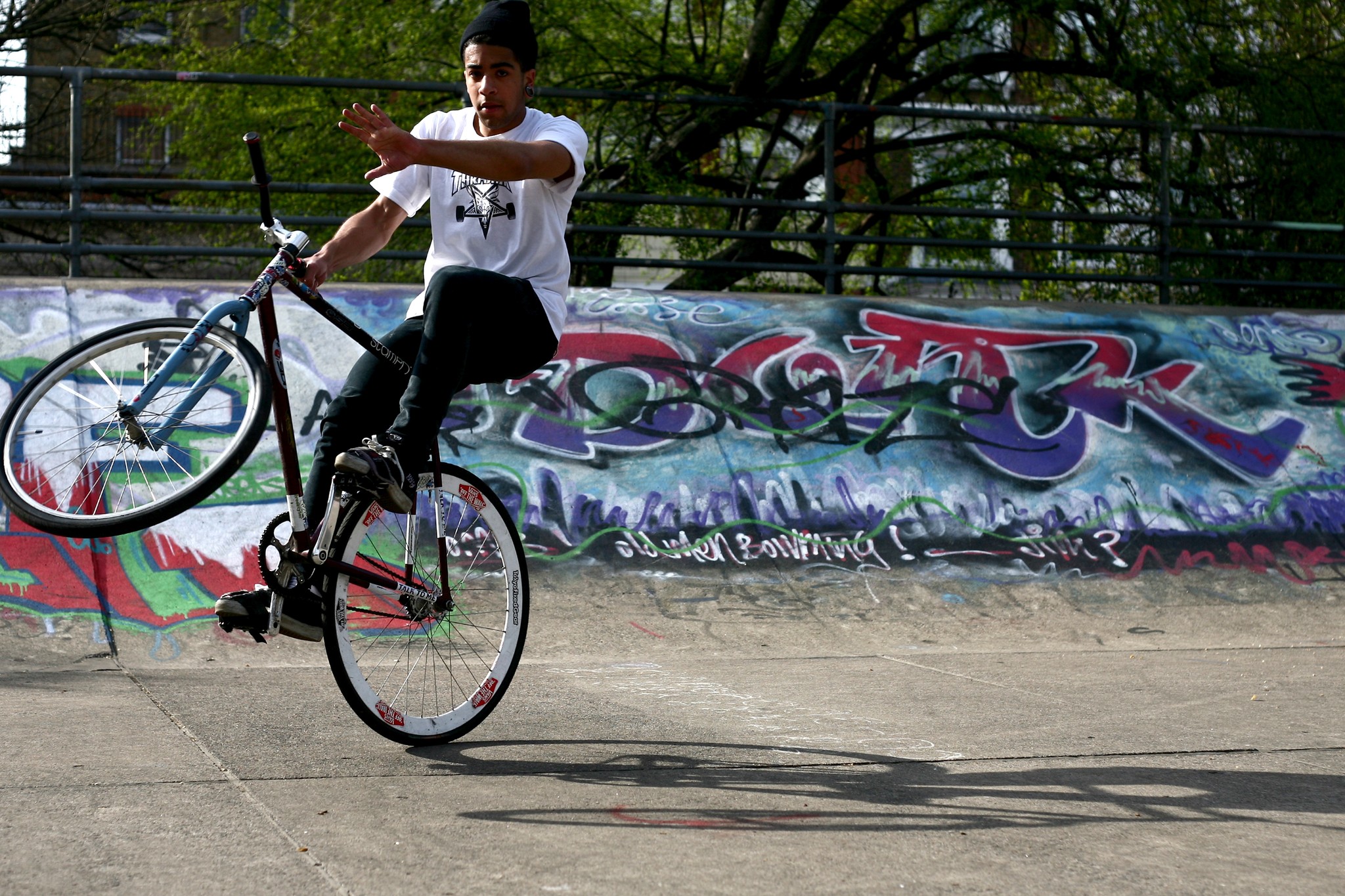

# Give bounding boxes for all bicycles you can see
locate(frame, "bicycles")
[0,127,531,745]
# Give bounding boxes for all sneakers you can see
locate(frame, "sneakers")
[214,582,322,642]
[334,432,420,515]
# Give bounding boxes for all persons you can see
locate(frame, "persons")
[214,0,587,642]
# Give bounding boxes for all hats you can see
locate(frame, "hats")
[459,0,538,74]
